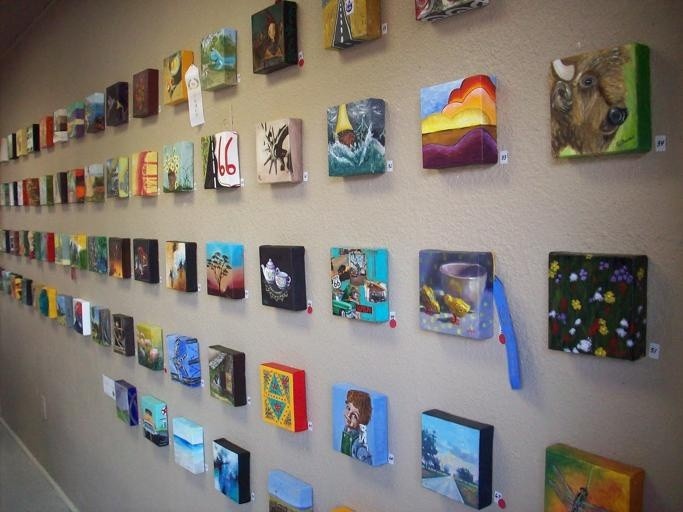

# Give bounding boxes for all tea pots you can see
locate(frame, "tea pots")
[261,258,280,285]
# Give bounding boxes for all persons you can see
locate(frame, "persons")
[262,14,283,59]
[341,389,373,466]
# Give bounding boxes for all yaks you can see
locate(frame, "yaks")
[549,43,632,157]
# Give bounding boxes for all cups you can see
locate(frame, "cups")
[274,272,291,289]
